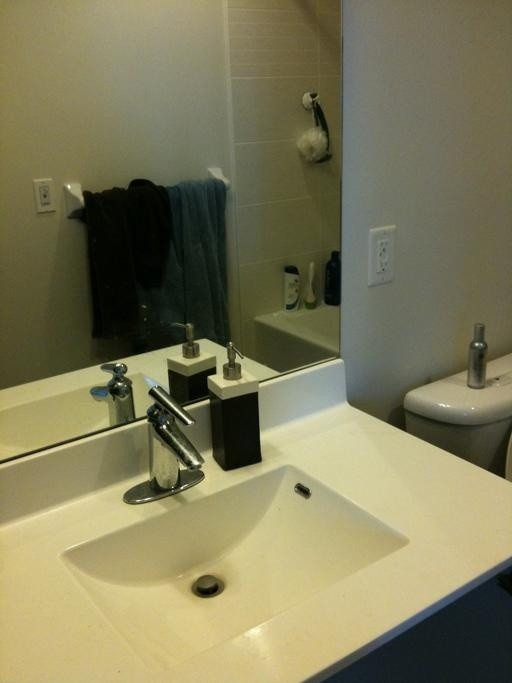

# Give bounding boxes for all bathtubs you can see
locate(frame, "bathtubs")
[254,298,340,374]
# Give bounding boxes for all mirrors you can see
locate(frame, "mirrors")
[0,0,340,465]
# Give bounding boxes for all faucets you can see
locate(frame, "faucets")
[146,386,204,493]
[89,363,135,428]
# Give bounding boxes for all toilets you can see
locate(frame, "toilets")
[404,352,512,483]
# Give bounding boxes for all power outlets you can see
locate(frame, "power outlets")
[368,225,395,286]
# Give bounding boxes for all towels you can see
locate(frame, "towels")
[33,178,56,214]
[164,177,232,346]
[69,178,170,339]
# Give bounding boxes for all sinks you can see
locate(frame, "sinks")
[59,465,409,675]
[0,372,155,464]
